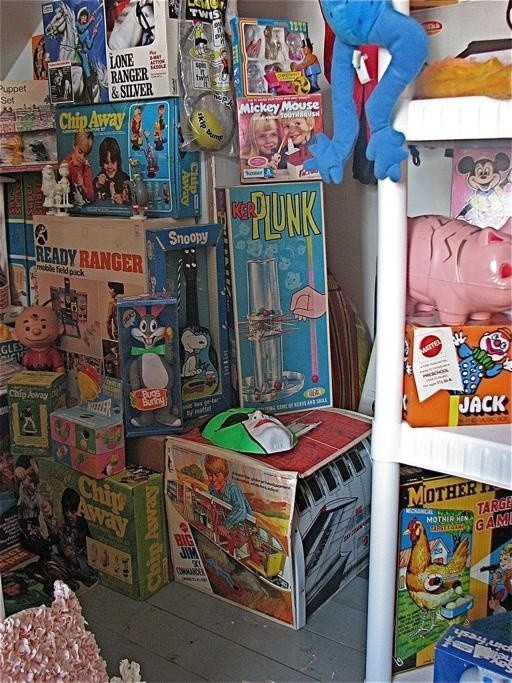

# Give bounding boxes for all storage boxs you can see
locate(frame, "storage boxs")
[0,0,377,630]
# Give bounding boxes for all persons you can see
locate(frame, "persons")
[14,452,59,559]
[57,485,91,568]
[203,455,254,539]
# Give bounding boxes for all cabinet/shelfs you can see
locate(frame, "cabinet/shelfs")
[359,0,511,682]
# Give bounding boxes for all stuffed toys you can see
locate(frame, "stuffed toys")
[301,0,431,186]
